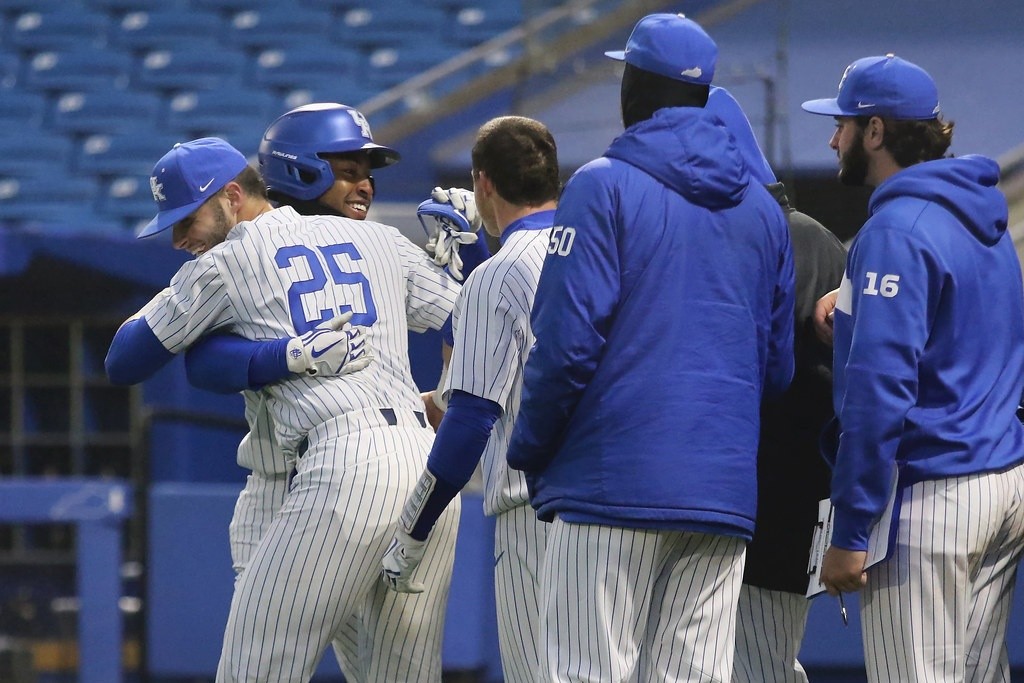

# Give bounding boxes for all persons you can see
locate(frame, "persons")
[811,54,1024,683]
[185,102,489,683]
[706,88,846,683]
[382,117,565,683]
[104,138,465,683]
[505,13,795,683]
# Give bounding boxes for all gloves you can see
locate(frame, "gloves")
[286,311,374,376]
[426,186,485,280]
[382,527,426,594]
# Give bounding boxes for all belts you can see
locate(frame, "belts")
[298,408,426,458]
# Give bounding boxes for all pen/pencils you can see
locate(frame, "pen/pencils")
[836,592,848,626]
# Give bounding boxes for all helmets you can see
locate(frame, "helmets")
[255,102,404,199]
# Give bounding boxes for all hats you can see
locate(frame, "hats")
[801,52,940,119]
[604,13,717,84]
[135,137,247,238]
